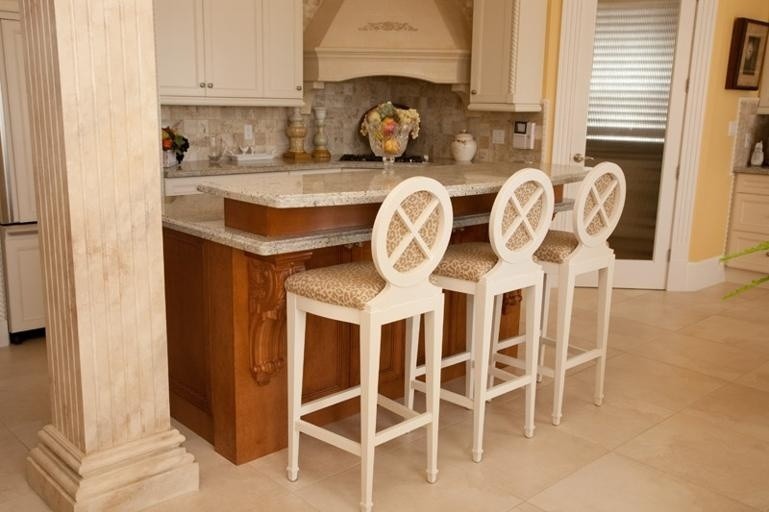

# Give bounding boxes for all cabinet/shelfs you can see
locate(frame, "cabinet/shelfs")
[2,224,46,343]
[0,9,39,223]
[154,1,304,106]
[468,0,549,112]
[726,174,769,275]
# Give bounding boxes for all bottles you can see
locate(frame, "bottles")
[749,140,766,167]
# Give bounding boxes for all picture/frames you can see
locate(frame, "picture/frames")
[726,19,767,90]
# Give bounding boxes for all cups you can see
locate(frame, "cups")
[207,135,224,163]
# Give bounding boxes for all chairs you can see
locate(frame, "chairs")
[404,167,555,463]
[486,162,627,426]
[285,175,454,512]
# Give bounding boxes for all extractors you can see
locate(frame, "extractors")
[303,1,470,94]
